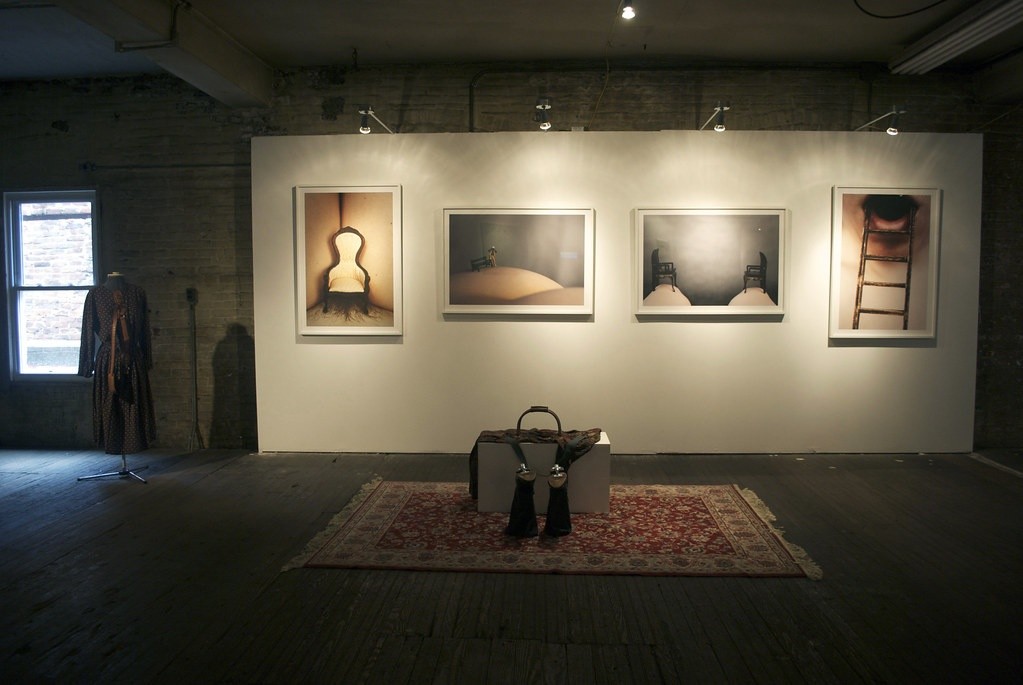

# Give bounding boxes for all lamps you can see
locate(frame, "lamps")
[535,98,552,130]
[700,100,731,132]
[886,0,1023,75]
[622,0,636,20]
[854,106,900,135]
[357,105,394,135]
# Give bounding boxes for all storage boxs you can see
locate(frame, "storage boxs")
[469,428,611,512]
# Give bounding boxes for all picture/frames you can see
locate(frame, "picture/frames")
[442,207,596,317]
[828,183,943,345]
[634,205,787,317]
[294,183,404,336]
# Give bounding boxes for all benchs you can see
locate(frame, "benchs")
[471,256,490,272]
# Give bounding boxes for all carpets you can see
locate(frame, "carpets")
[290,472,823,581]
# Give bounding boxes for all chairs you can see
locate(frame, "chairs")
[742,251,767,293]
[651,247,678,292]
[323,226,370,315]
